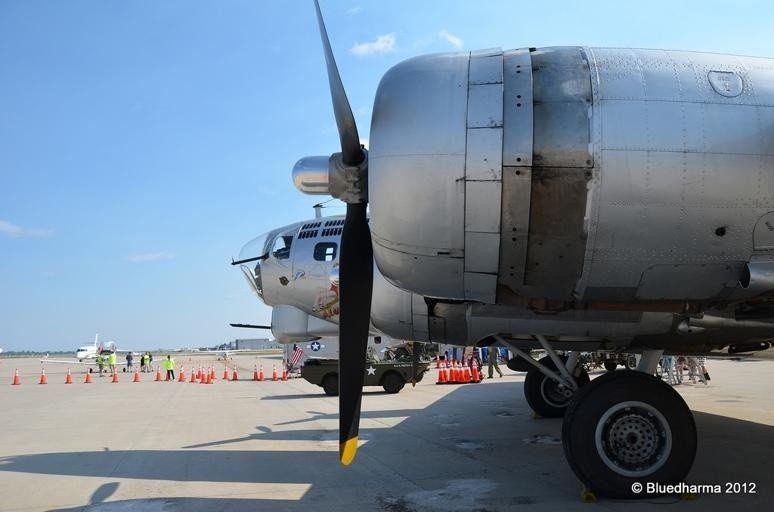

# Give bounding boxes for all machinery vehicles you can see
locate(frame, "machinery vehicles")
[301,340,426,394]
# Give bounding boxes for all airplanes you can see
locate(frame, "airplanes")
[75,333,116,363]
[198,348,252,360]
[222,0,774,499]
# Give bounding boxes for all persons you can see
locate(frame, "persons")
[96,348,153,378]
[160,354,175,381]
[664,355,708,386]
[485,346,503,379]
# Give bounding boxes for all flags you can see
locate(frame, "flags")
[291,343,304,367]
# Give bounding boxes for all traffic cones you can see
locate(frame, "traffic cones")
[435,355,485,385]
[11,365,288,385]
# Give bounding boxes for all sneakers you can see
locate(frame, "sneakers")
[667,375,711,386]
[486,373,504,378]
